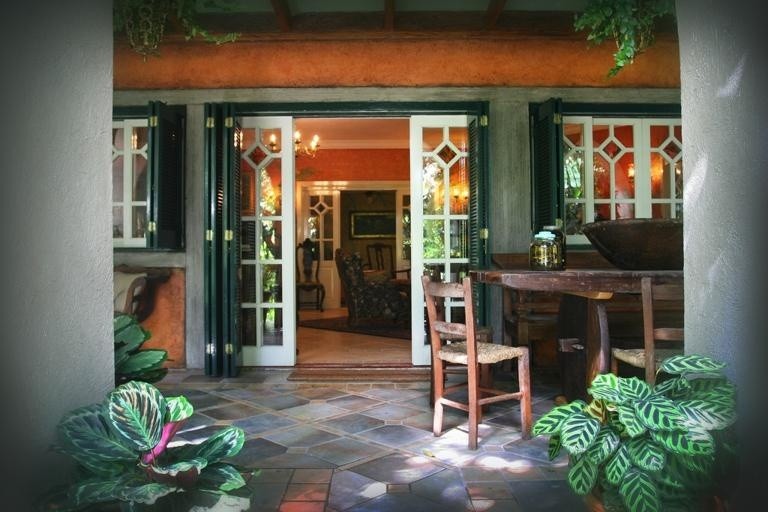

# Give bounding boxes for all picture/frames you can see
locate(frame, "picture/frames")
[348,210,396,240]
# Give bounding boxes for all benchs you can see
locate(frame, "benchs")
[492,249,685,390]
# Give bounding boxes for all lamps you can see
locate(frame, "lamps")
[267,132,321,159]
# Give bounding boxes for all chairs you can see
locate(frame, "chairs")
[423,266,532,449]
[295,237,327,312]
[609,278,685,385]
[114,262,172,323]
[333,243,411,330]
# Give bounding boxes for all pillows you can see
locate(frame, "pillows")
[114,271,148,315]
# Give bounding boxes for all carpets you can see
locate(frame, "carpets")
[298,316,410,340]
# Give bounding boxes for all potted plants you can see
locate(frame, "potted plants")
[531,354,744,512]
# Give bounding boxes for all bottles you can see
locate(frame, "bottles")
[527,225,567,271]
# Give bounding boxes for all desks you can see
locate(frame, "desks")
[467,268,685,404]
